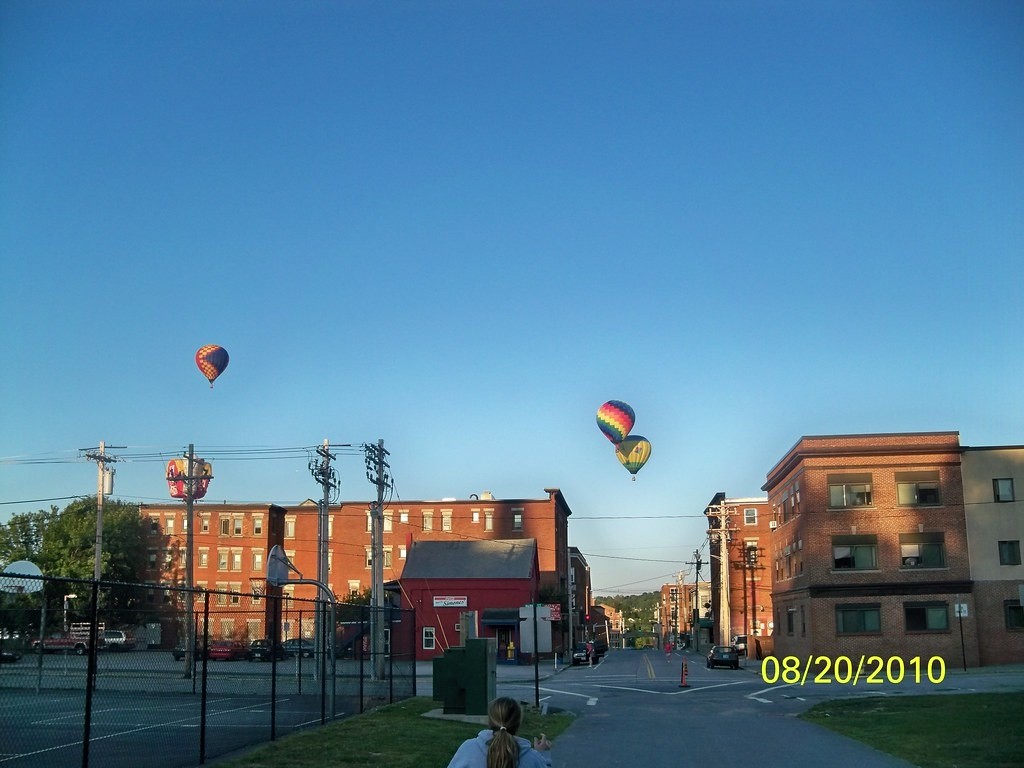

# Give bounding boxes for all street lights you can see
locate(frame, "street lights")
[63,594,78,676]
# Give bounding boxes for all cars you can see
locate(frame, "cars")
[173,638,204,661]
[572,641,605,664]
[281,639,315,658]
[248,639,284,661]
[207,639,251,662]
[0,649,22,662]
[706,645,739,669]
[731,636,748,654]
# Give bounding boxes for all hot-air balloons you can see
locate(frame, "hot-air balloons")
[615,434,652,482]
[596,400,635,453]
[195,344,230,388]
[166,453,213,504]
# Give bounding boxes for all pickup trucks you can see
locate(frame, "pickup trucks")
[31,629,106,656]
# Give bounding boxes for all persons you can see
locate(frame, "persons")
[586,641,594,668]
[664,640,672,662]
[447,696,553,768]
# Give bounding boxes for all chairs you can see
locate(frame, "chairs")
[715,650,720,653]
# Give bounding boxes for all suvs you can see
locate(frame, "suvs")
[97,629,137,653]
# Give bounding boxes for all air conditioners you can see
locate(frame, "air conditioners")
[769,521,777,529]
